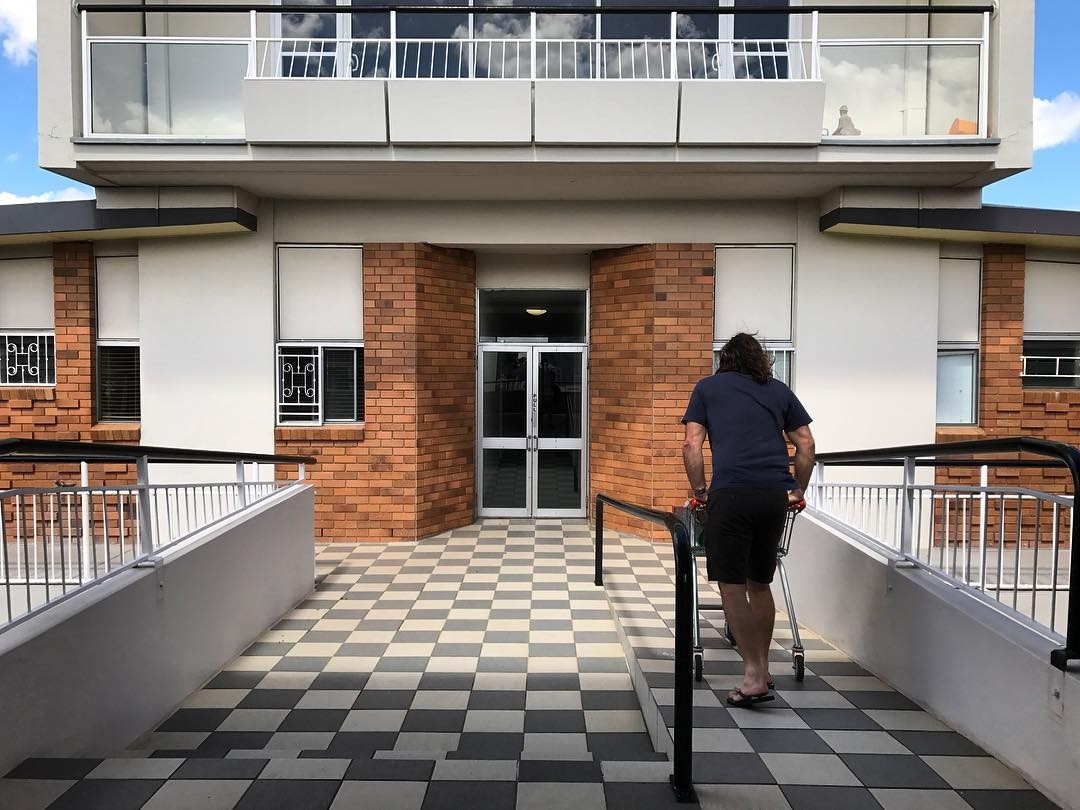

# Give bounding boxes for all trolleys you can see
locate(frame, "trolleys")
[671,497,807,683]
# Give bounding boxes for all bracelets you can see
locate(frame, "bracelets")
[692,485,706,494]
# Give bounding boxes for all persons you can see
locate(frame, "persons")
[681,333,816,708]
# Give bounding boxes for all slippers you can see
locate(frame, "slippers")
[767,672,775,690]
[727,689,775,706]
